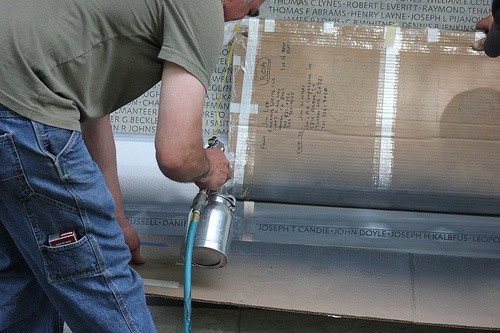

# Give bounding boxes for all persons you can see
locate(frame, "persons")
[472,0,500,58]
[2,1,266,333]
